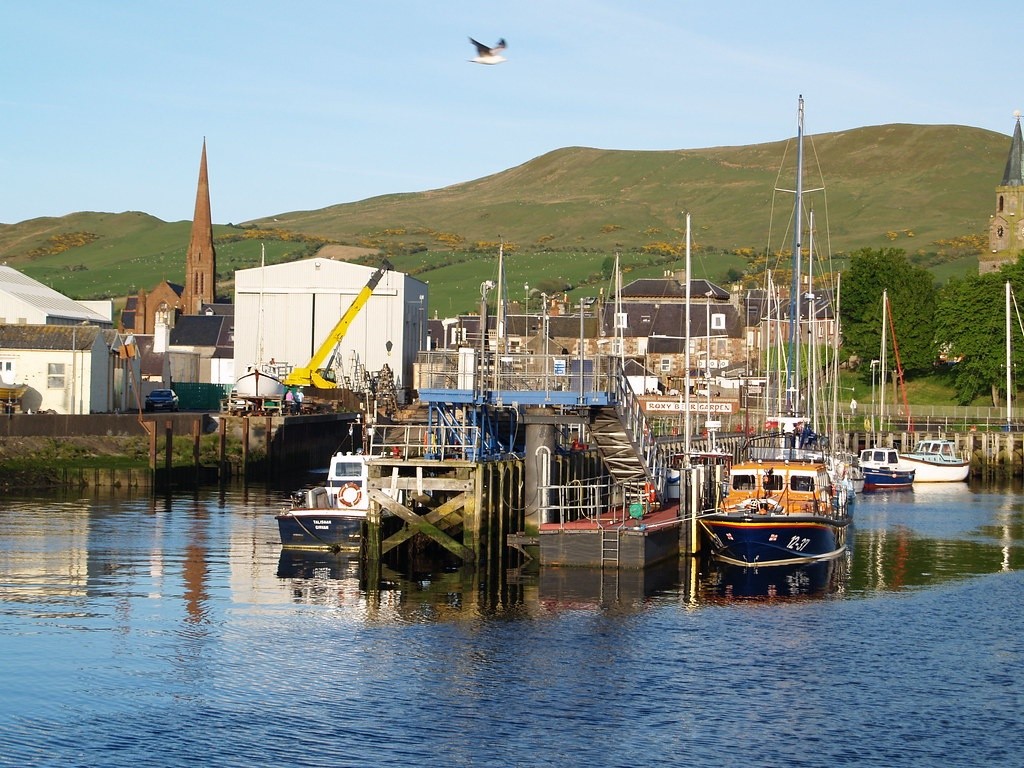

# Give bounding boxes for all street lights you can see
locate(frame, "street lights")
[71,321,89,415]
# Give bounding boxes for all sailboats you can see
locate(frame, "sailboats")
[230,243,298,400]
[274,93,868,576]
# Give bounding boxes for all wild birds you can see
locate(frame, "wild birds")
[466,36,507,65]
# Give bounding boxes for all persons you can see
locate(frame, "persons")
[270,358,276,371]
[294,389,304,415]
[710,390,721,397]
[285,388,298,414]
[763,476,769,490]
[431,430,437,453]
[646,388,655,394]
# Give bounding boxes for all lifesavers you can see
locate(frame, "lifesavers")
[646,483,656,503]
[339,482,362,508]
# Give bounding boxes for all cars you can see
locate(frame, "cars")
[143,388,180,413]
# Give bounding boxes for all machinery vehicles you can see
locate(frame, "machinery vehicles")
[281,259,394,417]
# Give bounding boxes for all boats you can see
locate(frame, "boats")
[857,448,917,491]
[897,441,972,482]
